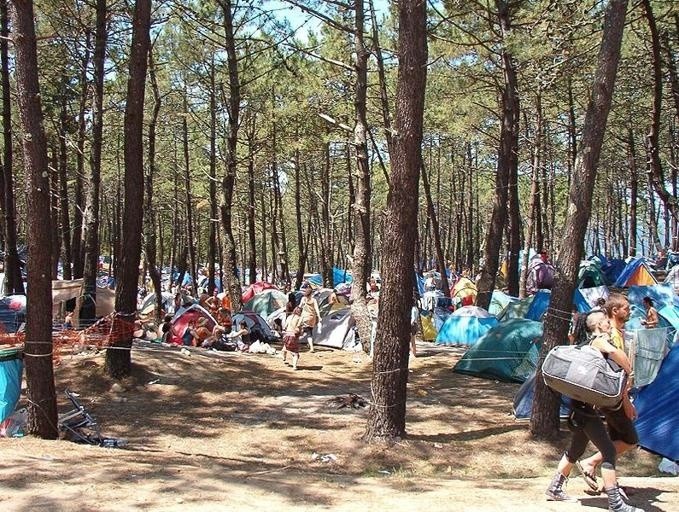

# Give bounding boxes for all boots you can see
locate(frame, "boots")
[604,487,645,512]
[545,470,579,503]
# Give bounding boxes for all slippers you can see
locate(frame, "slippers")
[574,459,599,491]
[603,483,636,495]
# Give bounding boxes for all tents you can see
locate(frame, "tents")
[1,240,677,462]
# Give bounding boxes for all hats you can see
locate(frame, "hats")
[196,317,209,327]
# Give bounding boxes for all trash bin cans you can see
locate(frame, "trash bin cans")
[0,344,24,430]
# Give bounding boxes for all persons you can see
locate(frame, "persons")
[541,294,660,512]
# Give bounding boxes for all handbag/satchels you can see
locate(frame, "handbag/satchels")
[541,344,627,412]
